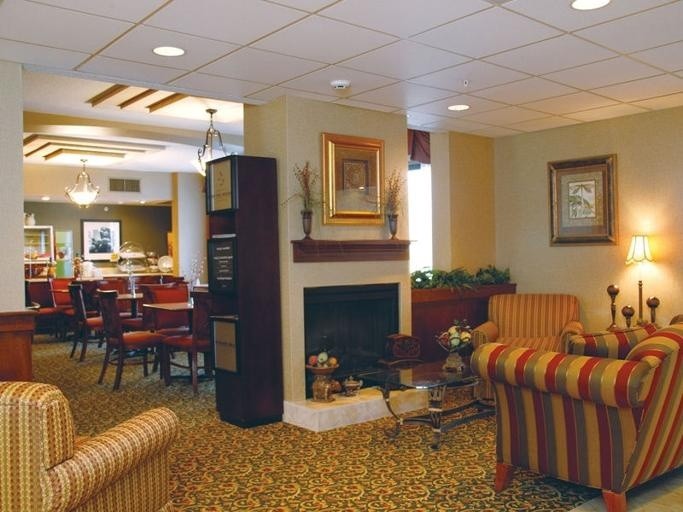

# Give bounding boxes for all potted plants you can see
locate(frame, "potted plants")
[279,160,332,240]
[382,167,408,239]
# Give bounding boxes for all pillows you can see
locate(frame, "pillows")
[570,323,662,358]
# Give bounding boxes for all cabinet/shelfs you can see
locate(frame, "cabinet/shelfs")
[24,226,54,277]
[206,154,284,428]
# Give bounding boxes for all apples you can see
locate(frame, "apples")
[441,325,470,346]
[308,352,337,368]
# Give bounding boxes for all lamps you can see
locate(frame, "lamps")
[192,109,236,176]
[625,234,655,323]
[64,158,100,209]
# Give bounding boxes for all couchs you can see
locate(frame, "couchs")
[0,380,180,512]
[470,293,584,407]
[469,314,683,512]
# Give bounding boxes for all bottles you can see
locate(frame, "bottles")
[73,252,83,279]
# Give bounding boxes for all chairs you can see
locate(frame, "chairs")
[51,273,208,390]
[25,281,60,341]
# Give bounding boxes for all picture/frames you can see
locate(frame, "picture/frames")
[546,153,619,245]
[320,132,386,225]
[80,219,123,263]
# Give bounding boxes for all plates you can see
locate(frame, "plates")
[157,255,174,274]
